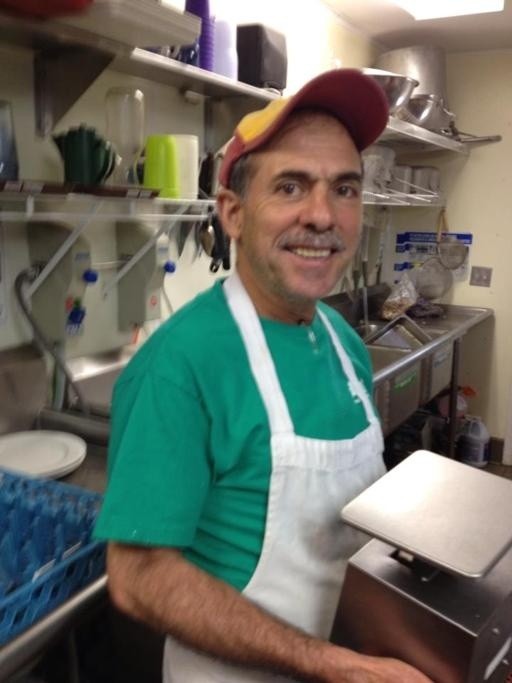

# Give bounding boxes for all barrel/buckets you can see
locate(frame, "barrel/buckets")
[461,416,491,467]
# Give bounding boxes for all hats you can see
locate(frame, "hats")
[216,67,389,187]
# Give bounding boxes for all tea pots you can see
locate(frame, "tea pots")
[50,123,118,187]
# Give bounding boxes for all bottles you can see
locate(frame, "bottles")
[183,0,216,71]
[105,86,147,186]
[0,100,21,188]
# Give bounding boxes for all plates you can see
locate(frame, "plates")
[0,430,88,481]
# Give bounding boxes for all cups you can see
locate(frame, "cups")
[138,132,201,200]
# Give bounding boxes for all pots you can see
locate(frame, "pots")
[375,38,448,132]
[358,146,443,207]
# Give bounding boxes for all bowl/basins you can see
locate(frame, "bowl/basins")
[364,75,422,116]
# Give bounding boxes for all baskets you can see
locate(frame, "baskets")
[1,470,107,646]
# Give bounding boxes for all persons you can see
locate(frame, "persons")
[91,65,430,682]
[342,570,463,682]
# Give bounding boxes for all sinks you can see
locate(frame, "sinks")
[369,346,420,432]
[349,319,454,407]
